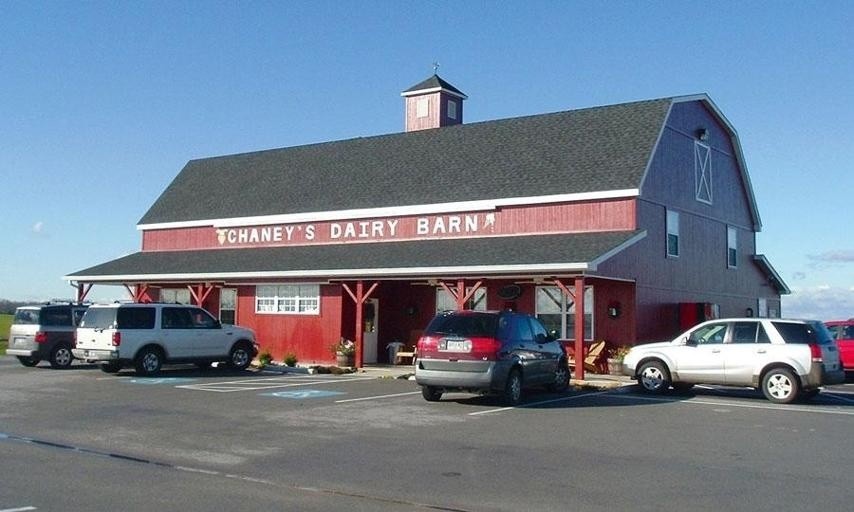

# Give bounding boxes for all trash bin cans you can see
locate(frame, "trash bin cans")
[386,342,406,365]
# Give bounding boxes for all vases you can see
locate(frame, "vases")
[607,358,622,375]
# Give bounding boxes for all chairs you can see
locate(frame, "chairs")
[395,329,424,366]
[735,328,744,342]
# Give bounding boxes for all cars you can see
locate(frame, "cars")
[5,300,259,376]
[415,309,571,404]
[623,317,854,404]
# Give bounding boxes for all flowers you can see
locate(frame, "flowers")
[607,344,628,360]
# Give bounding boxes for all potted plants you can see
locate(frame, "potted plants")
[331,337,355,366]
